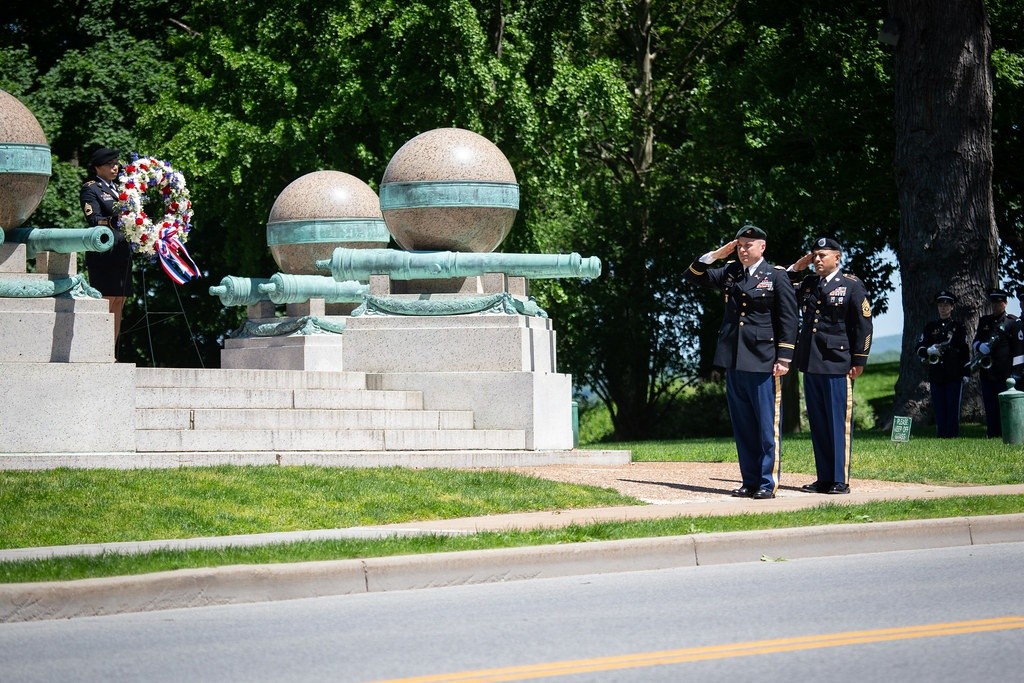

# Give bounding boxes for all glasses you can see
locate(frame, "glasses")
[108,159,117,165]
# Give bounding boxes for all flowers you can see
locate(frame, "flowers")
[119,153,193,263]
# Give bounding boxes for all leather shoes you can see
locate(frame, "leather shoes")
[732,486,758,497]
[753,489,775,498]
[801,480,835,493]
[827,483,850,494]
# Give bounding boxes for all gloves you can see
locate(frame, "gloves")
[927,344,942,356]
[979,342,990,355]
[1010,372,1021,379]
[963,376,970,384]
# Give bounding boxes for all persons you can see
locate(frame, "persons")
[787,238,873,494]
[918,291,970,438]
[81,149,164,362]
[683,226,797,499]
[972,288,1024,438]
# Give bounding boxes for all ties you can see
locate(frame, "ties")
[820,277,827,294]
[744,268,750,284]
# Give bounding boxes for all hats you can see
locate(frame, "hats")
[811,237,842,253]
[735,225,767,239]
[1016,289,1024,298]
[990,288,1012,301]
[91,148,120,166]
[935,290,957,304]
